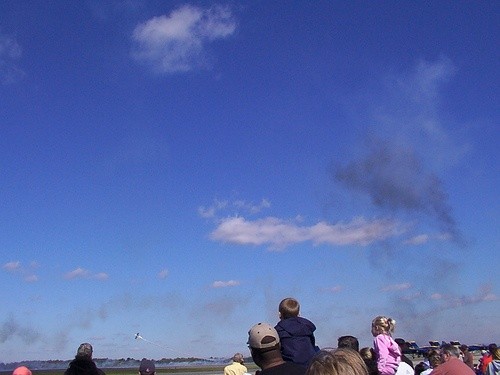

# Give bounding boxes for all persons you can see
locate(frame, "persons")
[364,315,402,375]
[224,353,247,375]
[306,347,370,375]
[394,338,500,375]
[274,297,320,375]
[63,342,106,375]
[138,357,156,375]
[12,365,32,375]
[246,322,307,375]
[338,336,372,359]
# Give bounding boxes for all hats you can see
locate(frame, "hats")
[13,366,33,375]
[247,322,280,348]
[139,360,156,375]
[77,342,92,357]
